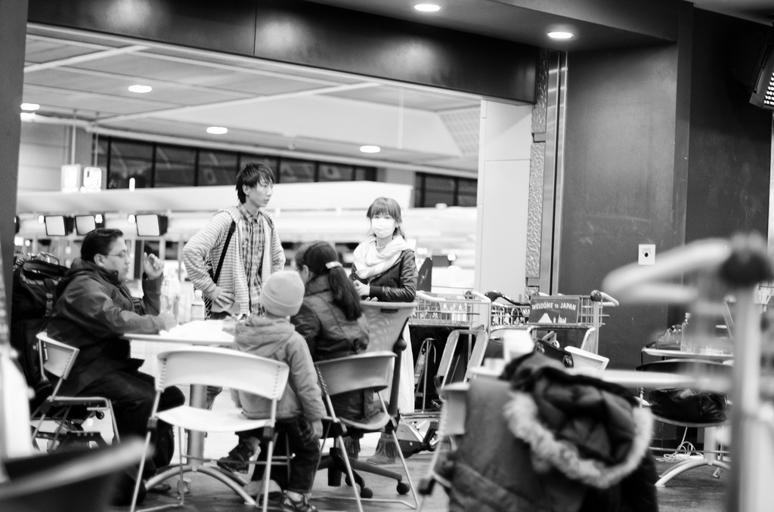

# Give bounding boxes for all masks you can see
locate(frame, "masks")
[371,218,396,239]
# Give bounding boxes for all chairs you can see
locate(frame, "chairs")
[360,288,744,509]
[33,328,122,455]
[302,349,422,511]
[129,344,292,511]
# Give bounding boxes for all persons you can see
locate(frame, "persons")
[210,237,373,474]
[38,228,180,476]
[213,266,328,512]
[339,195,419,315]
[180,162,287,324]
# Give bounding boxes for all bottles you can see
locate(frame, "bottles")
[680,312,693,353]
[189,290,205,322]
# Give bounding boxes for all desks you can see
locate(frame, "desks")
[141,317,309,509]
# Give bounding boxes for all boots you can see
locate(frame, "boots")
[343,436,395,464]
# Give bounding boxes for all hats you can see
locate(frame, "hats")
[260,269,305,316]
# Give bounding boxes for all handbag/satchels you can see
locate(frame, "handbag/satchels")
[202,260,213,319]
[649,387,726,423]
[11,260,70,349]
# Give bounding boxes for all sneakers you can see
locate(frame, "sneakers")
[280,495,318,511]
[216,457,249,474]
[237,480,281,497]
[143,473,171,492]
